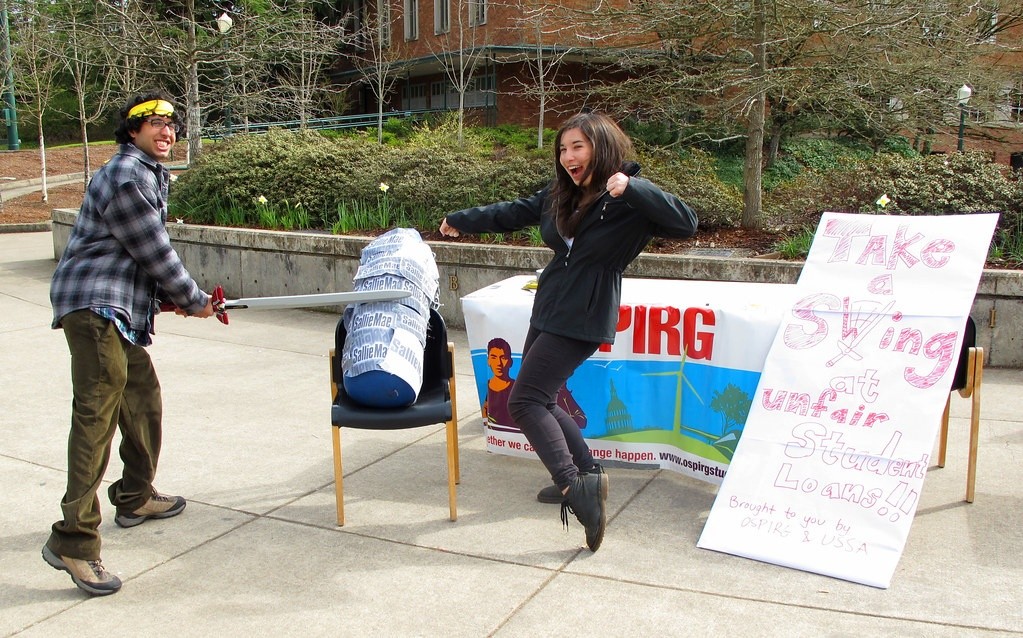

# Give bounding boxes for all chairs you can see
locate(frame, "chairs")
[938,315,982,502]
[326,308,459,527]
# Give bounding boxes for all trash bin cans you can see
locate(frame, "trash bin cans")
[1010,152,1023,173]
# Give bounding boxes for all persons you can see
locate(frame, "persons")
[42,97,213,594]
[440,113,697,552]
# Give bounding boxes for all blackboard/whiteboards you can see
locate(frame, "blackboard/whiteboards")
[698,211,998,589]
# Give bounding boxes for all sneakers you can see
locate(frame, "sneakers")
[537,465,606,503]
[113,487,186,527]
[560,473,609,551]
[42,532,122,596]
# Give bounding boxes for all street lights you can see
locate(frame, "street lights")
[956,80,973,153]
[216,12,233,130]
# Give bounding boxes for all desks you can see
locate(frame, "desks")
[459,273,796,488]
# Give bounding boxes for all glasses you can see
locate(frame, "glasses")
[142,118,180,133]
